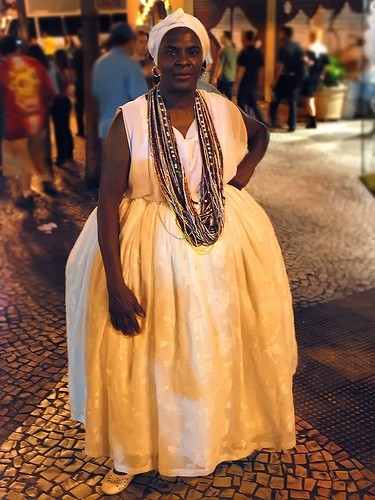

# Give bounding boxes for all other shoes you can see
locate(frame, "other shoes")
[63,158,86,173]
[41,180,59,197]
[101,468,133,495]
[15,194,38,212]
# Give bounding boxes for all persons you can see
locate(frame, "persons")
[269,24,304,131]
[89,21,147,192]
[0,17,82,206]
[297,27,329,129]
[132,29,160,88]
[356,29,375,138]
[235,30,268,125]
[212,30,237,100]
[65,8,299,493]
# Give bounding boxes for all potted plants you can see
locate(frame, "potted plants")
[315,58,348,120]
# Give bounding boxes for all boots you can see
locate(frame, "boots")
[306,115,316,128]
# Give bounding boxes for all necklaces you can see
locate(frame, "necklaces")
[146,85,227,253]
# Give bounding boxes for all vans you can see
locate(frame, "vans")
[4,8,129,60]
